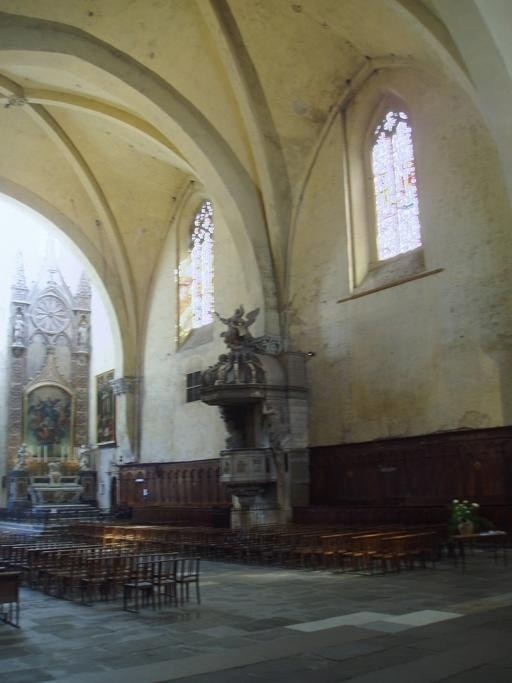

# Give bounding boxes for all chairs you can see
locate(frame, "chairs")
[1,521,507,631]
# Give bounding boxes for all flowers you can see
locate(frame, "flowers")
[452,498,480,524]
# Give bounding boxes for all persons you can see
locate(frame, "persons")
[218,304,244,343]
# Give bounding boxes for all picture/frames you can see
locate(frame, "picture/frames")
[95,369,116,447]
